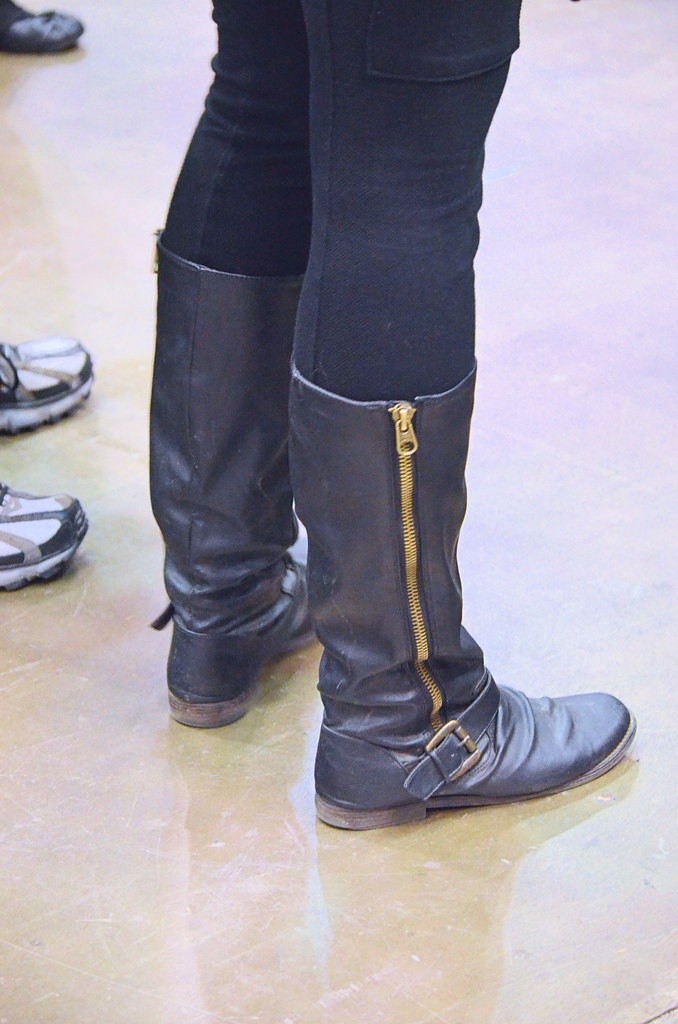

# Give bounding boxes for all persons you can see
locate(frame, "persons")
[154,1,636,832]
[0,0,95,588]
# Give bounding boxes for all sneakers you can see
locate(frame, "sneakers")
[0,481,89,593]
[0,334,94,436]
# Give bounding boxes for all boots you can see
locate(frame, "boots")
[148,233,322,728]
[288,351,637,831]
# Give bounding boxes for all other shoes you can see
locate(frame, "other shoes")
[0,12,85,55]
